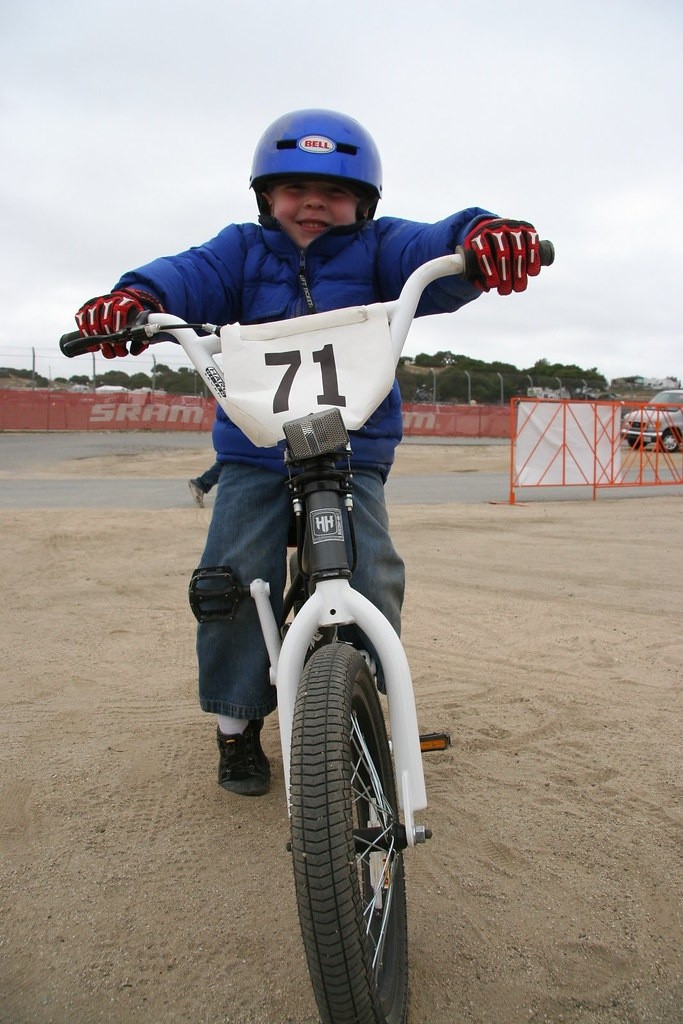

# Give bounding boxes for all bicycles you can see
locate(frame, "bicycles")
[60,237,556,1023]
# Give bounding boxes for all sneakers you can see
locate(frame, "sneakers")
[216,714,270,796]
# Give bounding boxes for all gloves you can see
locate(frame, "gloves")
[75,287,166,359]
[463,218,540,296]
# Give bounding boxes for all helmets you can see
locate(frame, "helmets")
[248,106,383,200]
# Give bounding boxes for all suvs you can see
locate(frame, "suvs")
[621,390,683,452]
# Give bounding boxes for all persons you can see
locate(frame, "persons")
[73,104,542,795]
